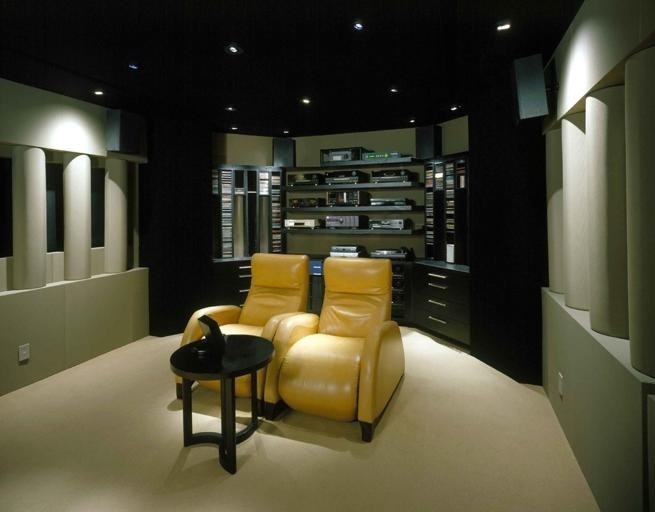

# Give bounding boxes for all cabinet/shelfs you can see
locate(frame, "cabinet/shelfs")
[411,261,472,345]
[286,160,423,236]
[389,256,414,329]
[424,148,473,259]
[212,164,284,258]
[214,260,252,309]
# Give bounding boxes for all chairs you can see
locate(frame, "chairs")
[173,250,313,424]
[266,256,410,441]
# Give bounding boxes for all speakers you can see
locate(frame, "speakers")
[415,124,442,160]
[512,49,558,126]
[104,109,145,156]
[272,139,296,168]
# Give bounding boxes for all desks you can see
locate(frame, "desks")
[169,333,277,476]
